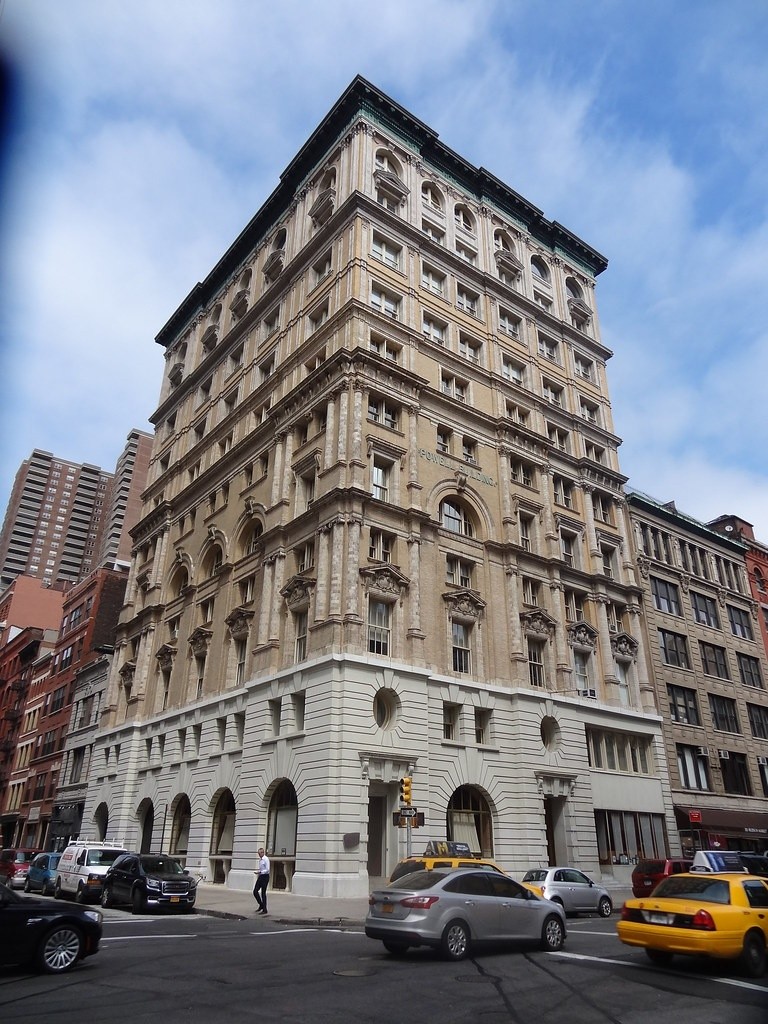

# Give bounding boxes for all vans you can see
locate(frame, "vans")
[0,849,47,890]
[54,835,130,906]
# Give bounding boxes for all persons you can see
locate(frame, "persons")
[252,848,270,915]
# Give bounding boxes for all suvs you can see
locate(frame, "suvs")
[737,850,768,879]
[101,853,196,915]
[24,852,64,897]
[631,859,694,899]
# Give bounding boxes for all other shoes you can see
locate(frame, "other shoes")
[255,906,264,912]
[259,911,267,915]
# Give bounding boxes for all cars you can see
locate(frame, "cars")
[387,840,544,898]
[364,867,567,962]
[521,866,613,917]
[616,851,768,977]
[0,882,104,974]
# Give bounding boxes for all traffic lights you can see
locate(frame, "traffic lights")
[399,776,412,803]
